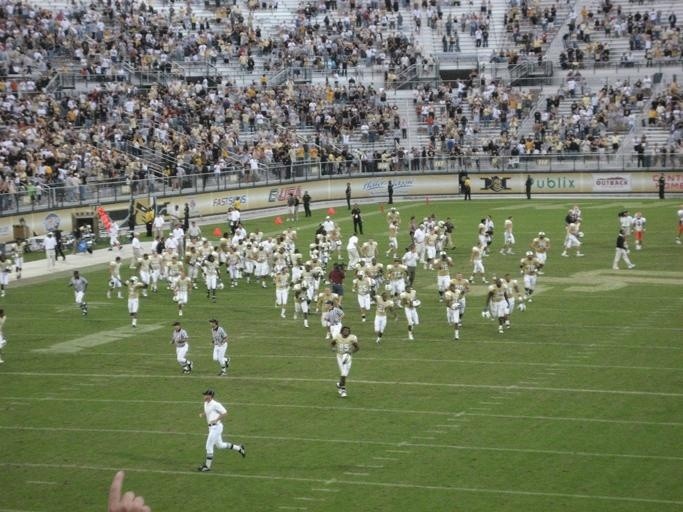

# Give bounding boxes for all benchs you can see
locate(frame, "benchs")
[0,0,681,213]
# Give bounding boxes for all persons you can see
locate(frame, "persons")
[614,209,647,269]
[1,202,224,327]
[168,322,194,375]
[209,318,232,374]
[676,209,683,244]
[1,0,683,213]
[328,327,359,396]
[218,181,582,341]
[198,390,246,473]
[109,471,154,512]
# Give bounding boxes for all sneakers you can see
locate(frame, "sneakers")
[575,252,585,257]
[226,357,232,367]
[127,208,551,347]
[628,264,636,269]
[105,291,111,299]
[336,380,348,399]
[182,360,195,375]
[116,291,125,300]
[217,372,228,376]
[238,445,247,458]
[612,268,620,270]
[197,465,210,473]
[560,251,571,258]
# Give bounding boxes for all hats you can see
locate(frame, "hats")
[202,389,216,396]
